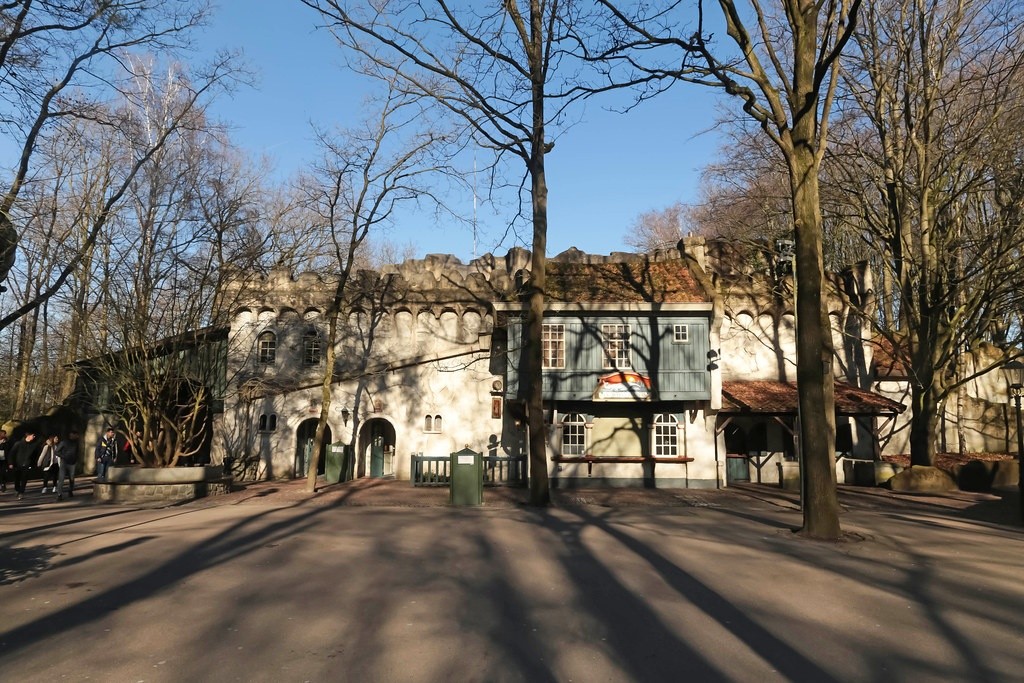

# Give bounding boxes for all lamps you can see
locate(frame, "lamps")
[341,406,351,426]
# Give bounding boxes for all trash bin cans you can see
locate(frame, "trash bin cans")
[325,439,350,483]
[449,445,485,510]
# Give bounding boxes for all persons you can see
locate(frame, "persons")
[0,430,9,491]
[55,430,80,502]
[125,428,145,464]
[94,428,118,478]
[9,431,38,501]
[39,435,62,494]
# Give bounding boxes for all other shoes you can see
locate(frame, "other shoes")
[42,487,47,493]
[52,486,56,492]
[57,495,62,501]
[1,484,6,492]
[68,490,73,497]
[15,491,19,496]
[18,493,24,500]
